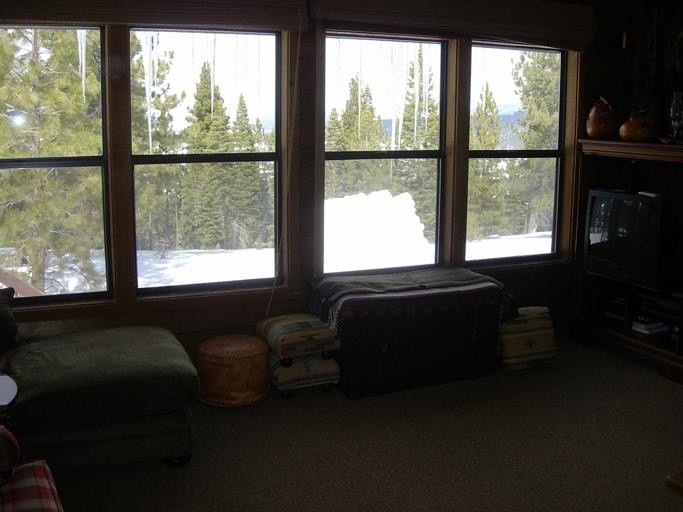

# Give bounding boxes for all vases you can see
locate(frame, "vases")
[584,96,654,143]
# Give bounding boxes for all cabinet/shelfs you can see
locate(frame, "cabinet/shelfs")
[575,274,683,384]
[334,305,500,401]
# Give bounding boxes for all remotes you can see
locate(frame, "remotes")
[638,191,660,198]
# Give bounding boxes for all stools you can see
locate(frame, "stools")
[196,310,338,408]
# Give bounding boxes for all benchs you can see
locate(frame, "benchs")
[0,323,201,467]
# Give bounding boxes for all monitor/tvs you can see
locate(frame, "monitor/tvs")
[582,187,683,294]
